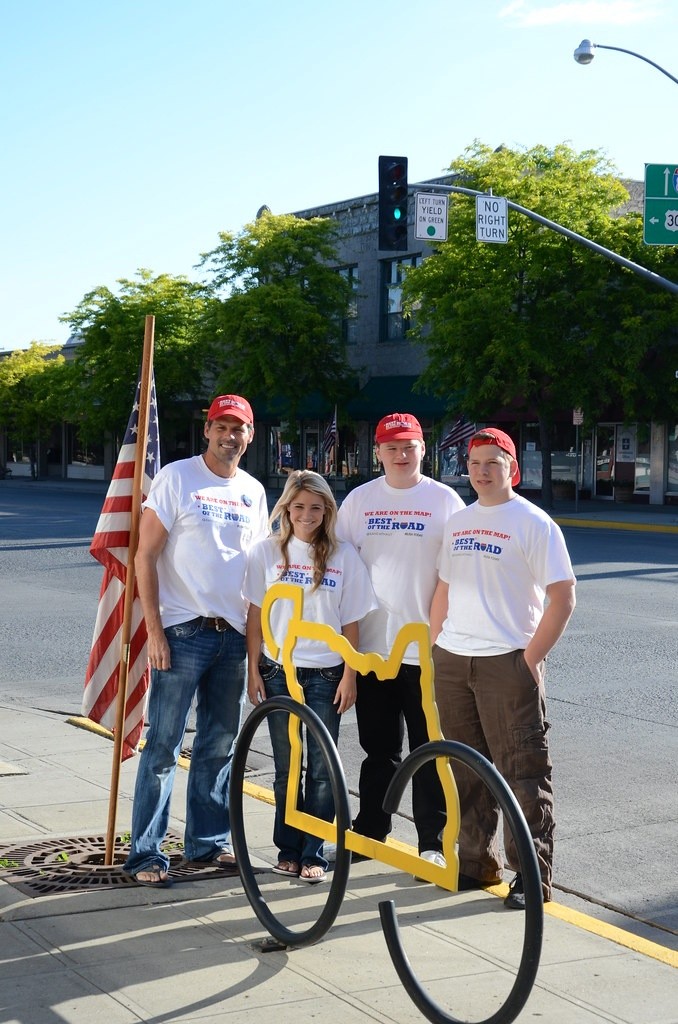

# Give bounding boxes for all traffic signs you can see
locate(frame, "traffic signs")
[642,162,678,246]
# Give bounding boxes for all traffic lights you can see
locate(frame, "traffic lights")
[377,154,409,251]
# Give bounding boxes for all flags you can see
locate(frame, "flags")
[438,414,475,451]
[80,364,160,764]
[322,413,336,454]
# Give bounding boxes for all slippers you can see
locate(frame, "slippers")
[272,860,298,877]
[212,848,237,867]
[133,862,173,888]
[299,864,328,882]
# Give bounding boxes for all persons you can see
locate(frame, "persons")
[121,394,273,889]
[239,470,379,885]
[320,413,466,884]
[428,428,577,911]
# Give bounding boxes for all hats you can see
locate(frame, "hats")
[207,394,254,424]
[376,413,423,444]
[468,428,521,487]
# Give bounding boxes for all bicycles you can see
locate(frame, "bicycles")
[226,578,546,1024]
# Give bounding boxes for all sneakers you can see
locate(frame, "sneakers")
[504,872,526,908]
[323,844,337,861]
[458,873,503,891]
[413,850,447,886]
